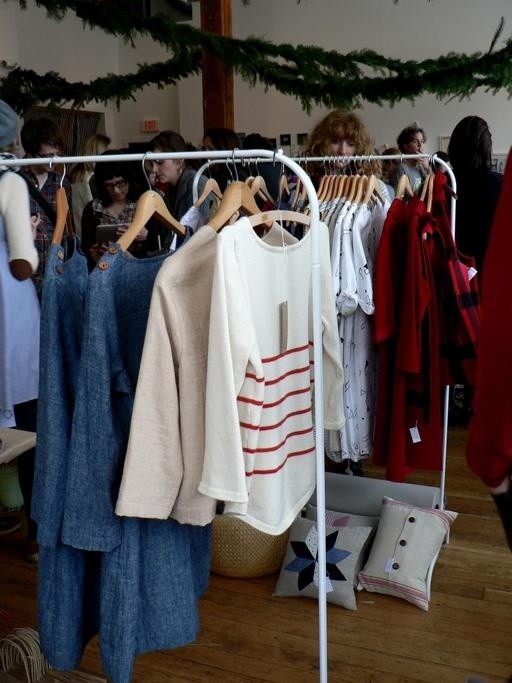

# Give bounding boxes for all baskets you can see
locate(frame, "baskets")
[210,513,289,579]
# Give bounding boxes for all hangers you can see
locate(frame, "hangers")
[44,156,75,266]
[160,149,300,275]
[180,161,224,223]
[178,152,437,253]
[94,150,187,273]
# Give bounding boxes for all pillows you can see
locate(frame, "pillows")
[306,503,381,588]
[357,496,459,611]
[272,516,373,611]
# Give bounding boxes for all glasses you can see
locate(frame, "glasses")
[104,180,127,190]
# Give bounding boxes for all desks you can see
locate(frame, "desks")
[0,427,37,467]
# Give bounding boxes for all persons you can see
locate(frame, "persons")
[2,101,502,425]
[0,166,41,536]
[464,143,512,552]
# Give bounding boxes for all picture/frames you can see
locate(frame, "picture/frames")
[491,153,508,175]
[439,136,451,154]
